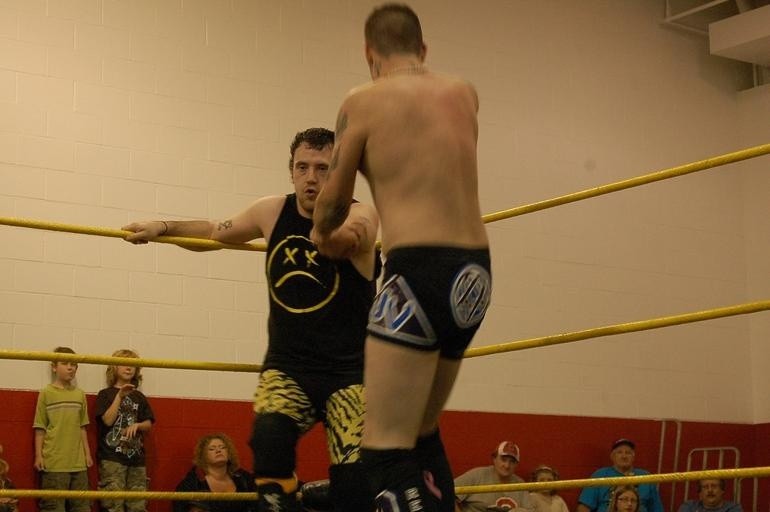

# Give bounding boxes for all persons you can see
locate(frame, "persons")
[455,441,531,511]
[95,349,155,511]
[576,439,663,512]
[170,432,258,511]
[680,477,740,511]
[33,348,92,511]
[122,127,379,512]
[529,467,567,511]
[310,3,493,510]
[610,484,641,511]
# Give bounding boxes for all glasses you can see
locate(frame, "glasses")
[698,481,719,491]
[617,494,638,504]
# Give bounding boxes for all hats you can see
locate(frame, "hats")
[489,440,521,464]
[609,437,638,452]
